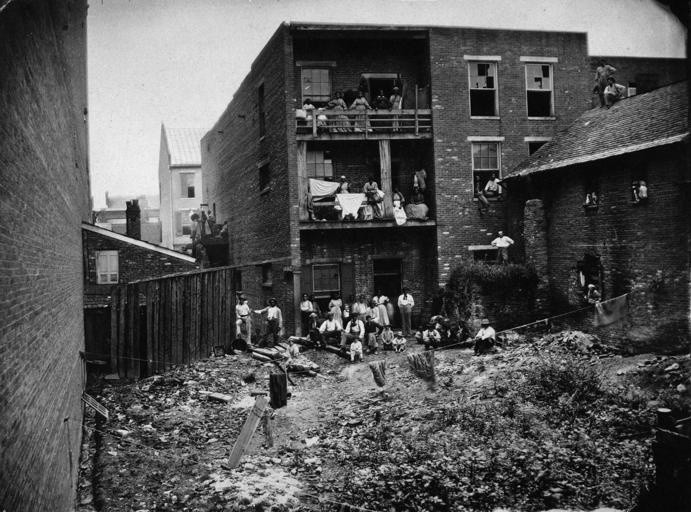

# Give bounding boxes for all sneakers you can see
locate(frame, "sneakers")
[367,349,370,352]
[473,352,481,356]
[375,351,379,355]
[396,349,401,353]
[361,359,364,362]
[352,361,355,363]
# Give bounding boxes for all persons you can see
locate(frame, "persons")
[593,57,617,109]
[490,230,515,264]
[232,292,253,349]
[602,76,626,110]
[481,170,502,200]
[252,295,285,348]
[473,317,496,358]
[300,286,452,361]
[285,337,300,368]
[300,84,424,205]
[586,283,602,311]
[473,175,491,216]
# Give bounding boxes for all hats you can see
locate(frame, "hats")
[393,86,399,90]
[427,323,433,326]
[381,325,392,328]
[397,331,402,336]
[267,297,277,305]
[498,231,503,234]
[239,294,247,299]
[481,319,489,325]
[402,286,410,291]
[341,175,345,178]
[350,312,359,317]
